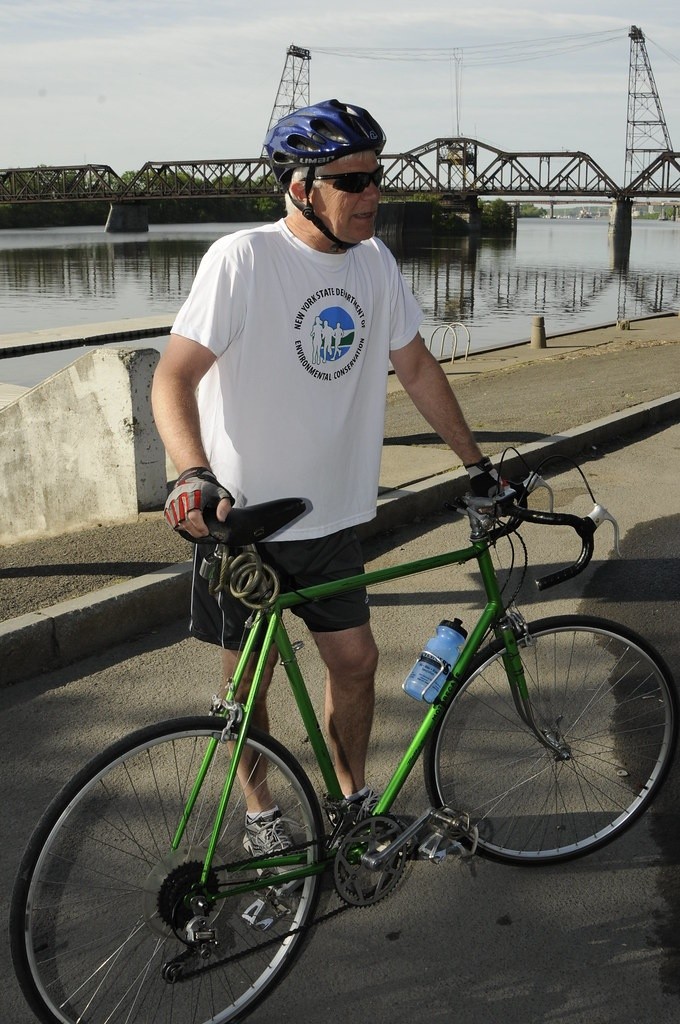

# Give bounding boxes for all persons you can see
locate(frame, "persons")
[150,99,508,892]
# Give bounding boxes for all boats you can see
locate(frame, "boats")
[579,208,593,218]
[657,208,671,220]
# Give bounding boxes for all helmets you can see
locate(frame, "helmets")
[262,99,387,192]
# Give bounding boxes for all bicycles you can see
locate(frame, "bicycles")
[10,448,679,1023]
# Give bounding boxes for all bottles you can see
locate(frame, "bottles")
[405,617,468,702]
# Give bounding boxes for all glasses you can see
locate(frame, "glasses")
[298,165,385,193]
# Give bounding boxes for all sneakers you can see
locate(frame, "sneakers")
[243,809,308,892]
[329,789,420,857]
